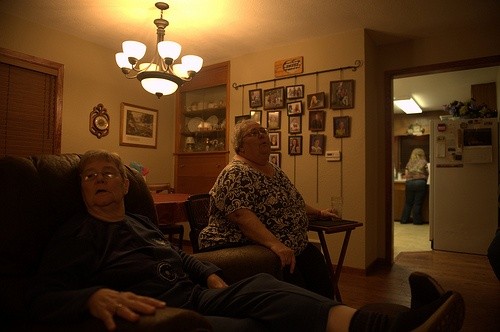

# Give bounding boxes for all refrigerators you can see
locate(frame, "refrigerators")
[428,118,498,256]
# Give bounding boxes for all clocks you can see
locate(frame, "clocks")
[89,103,110,138]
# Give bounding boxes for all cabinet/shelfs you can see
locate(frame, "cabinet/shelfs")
[393,181,429,222]
[173,60,231,194]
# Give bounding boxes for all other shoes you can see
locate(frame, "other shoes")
[408,272,445,310]
[394,290,465,332]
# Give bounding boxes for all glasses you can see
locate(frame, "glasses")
[79,168,123,182]
[242,129,269,138]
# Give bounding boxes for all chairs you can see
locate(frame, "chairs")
[185,193,210,254]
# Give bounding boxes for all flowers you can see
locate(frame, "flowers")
[443,98,498,119]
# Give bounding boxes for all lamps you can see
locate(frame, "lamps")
[114,2,203,100]
[393,96,422,114]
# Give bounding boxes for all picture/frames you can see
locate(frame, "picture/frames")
[119,102,159,149]
[234,79,355,168]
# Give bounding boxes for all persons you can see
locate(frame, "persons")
[289,87,301,96]
[199,120,342,301]
[251,91,256,104]
[336,121,346,137]
[291,118,299,129]
[271,157,277,165]
[265,92,284,106]
[290,139,300,154]
[310,96,319,107]
[311,138,323,153]
[0,150,466,332]
[289,104,300,112]
[312,113,323,128]
[400,148,429,224]
[271,136,276,145]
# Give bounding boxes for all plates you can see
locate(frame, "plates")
[182,115,226,130]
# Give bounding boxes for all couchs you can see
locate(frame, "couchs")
[0,153,268,332]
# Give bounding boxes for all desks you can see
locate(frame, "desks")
[307,217,363,305]
[148,191,193,250]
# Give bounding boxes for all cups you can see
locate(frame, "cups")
[330,197,343,222]
